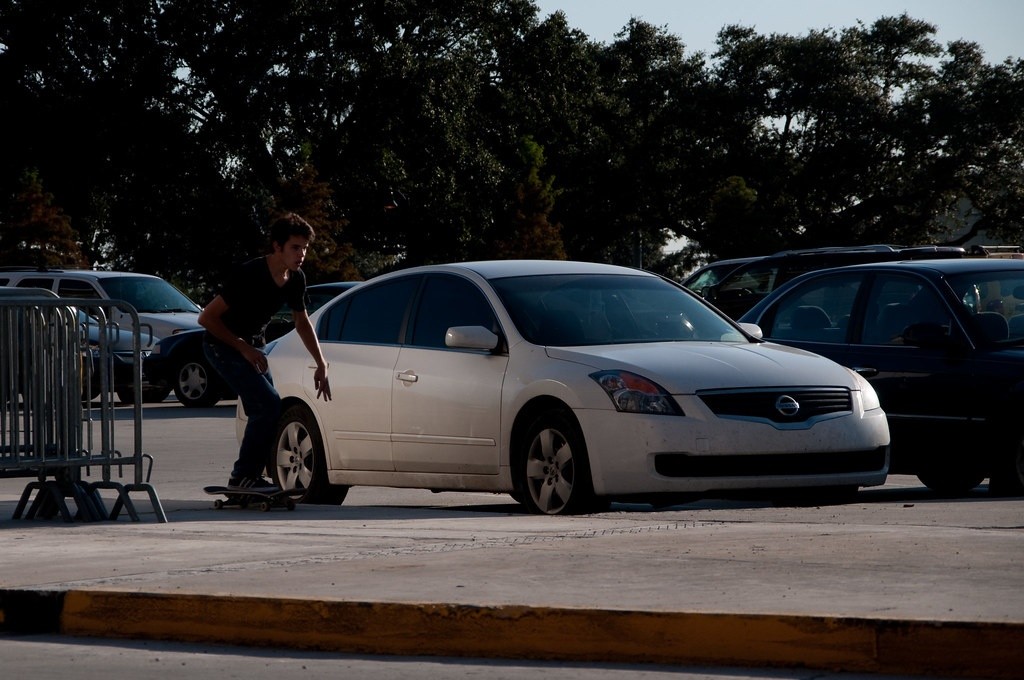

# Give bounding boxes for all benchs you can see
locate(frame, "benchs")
[774,306,844,341]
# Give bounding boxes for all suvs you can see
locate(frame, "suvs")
[0,265,205,339]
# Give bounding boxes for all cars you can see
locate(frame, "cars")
[143,281,364,408]
[0,295,175,408]
[651,243,1023,499]
[261,258,893,516]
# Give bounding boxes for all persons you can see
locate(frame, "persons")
[197,212,332,507]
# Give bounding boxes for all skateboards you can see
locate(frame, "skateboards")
[203,485,295,512]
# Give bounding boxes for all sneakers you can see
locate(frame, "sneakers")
[228,472,278,492]
[233,492,271,505]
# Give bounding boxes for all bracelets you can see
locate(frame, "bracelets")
[241,346,256,354]
[232,337,245,345]
